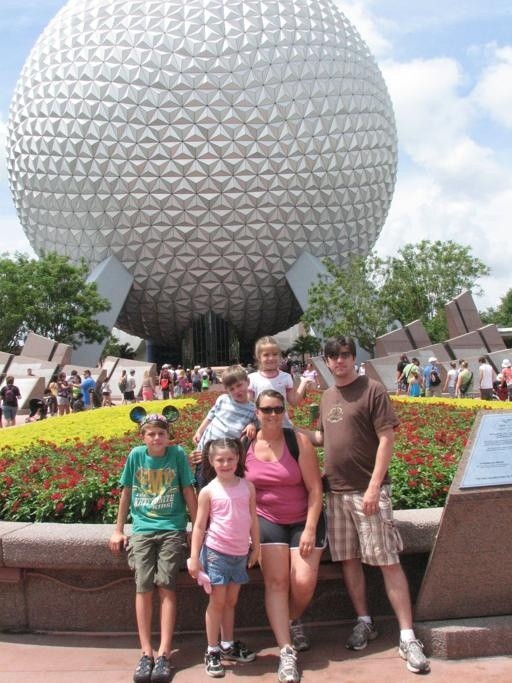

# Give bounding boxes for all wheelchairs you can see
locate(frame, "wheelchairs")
[490,386,508,401]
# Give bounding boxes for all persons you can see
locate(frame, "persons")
[281,354,318,388]
[247,364,255,375]
[396,353,510,401]
[187,361,263,465]
[355,363,366,374]
[120,363,218,405]
[238,390,328,681]
[107,412,198,682]
[244,335,318,428]
[189,434,262,678]
[293,335,433,676]
[0,368,113,425]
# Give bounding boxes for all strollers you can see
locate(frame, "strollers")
[25,398,47,423]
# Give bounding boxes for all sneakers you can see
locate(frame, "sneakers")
[399,636,432,674]
[204,647,225,679]
[219,642,257,663]
[287,619,311,651]
[134,655,152,682]
[151,656,174,682]
[346,616,379,651]
[278,645,300,683]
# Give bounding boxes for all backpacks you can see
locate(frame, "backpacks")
[119,377,128,392]
[5,385,15,401]
[161,371,171,390]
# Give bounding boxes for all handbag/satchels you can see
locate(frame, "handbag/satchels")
[429,370,442,385]
[459,382,469,392]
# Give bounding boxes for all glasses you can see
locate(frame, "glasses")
[327,351,353,360]
[258,406,284,414]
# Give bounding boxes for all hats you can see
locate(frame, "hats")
[401,355,437,363]
[496,358,511,381]
[81,370,91,375]
[162,364,201,370]
